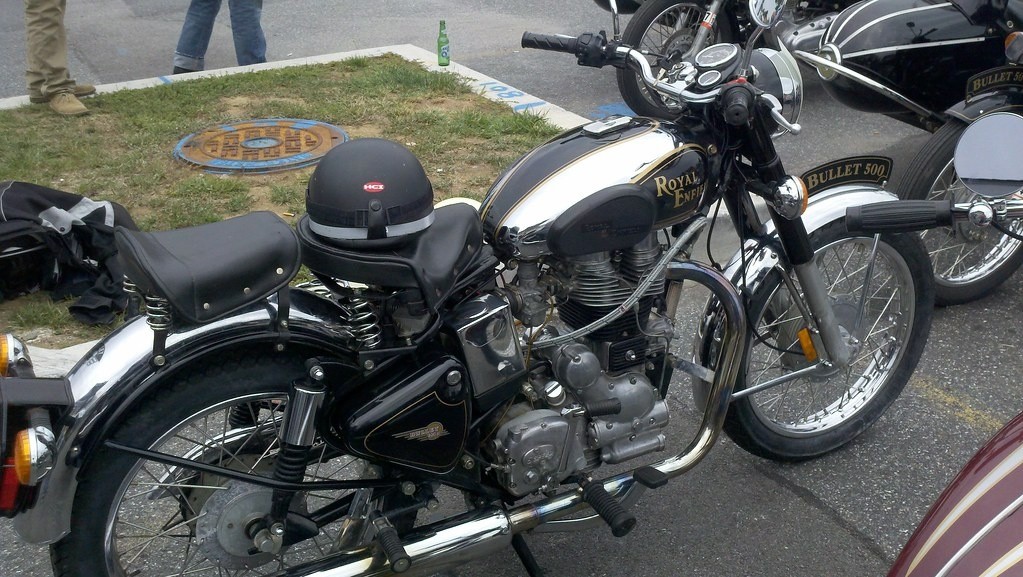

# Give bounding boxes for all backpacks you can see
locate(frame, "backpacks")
[0,229,83,303]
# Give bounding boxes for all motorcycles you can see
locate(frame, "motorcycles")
[0,0,945,577]
[593,0,1023,308]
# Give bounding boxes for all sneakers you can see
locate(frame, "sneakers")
[30,82,96,103]
[49,91,92,117]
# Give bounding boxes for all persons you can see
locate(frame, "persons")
[23,0,96,116]
[174,0,267,74]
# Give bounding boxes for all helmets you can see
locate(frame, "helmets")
[306,137,435,253]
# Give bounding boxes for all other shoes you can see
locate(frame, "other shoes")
[173,66,193,74]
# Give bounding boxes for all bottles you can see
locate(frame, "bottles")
[437,21,450,67]
